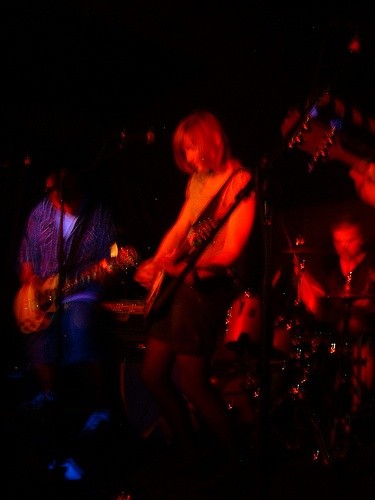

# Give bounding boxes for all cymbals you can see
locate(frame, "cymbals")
[318,291,375,301]
[277,246,335,256]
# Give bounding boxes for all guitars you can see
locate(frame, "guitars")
[141,215,220,314]
[280,107,375,178]
[12,245,139,334]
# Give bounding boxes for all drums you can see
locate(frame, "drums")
[269,315,308,349]
[220,287,264,355]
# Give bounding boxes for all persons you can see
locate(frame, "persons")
[134,112,257,459]
[330,220,375,306]
[21,167,118,406]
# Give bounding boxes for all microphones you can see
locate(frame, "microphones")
[120,130,156,142]
